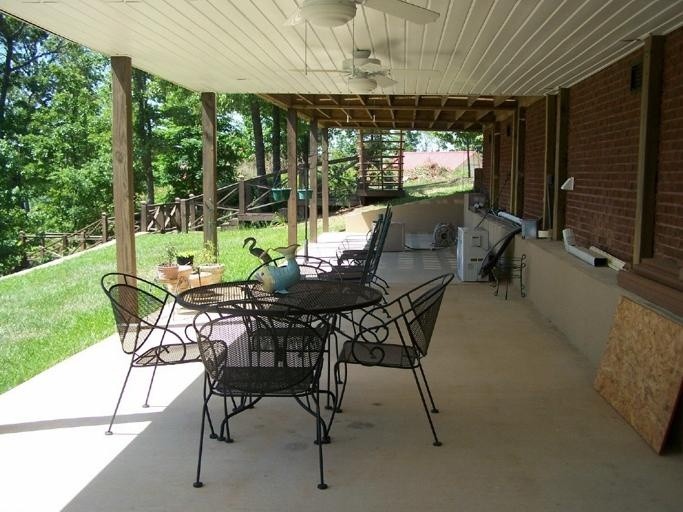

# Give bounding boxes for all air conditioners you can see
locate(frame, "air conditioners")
[457,226,490,282]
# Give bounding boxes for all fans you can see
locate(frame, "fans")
[288,1,441,87]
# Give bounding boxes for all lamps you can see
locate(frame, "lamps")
[347,79,377,93]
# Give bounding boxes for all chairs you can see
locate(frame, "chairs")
[192,300,333,491]
[318,202,396,326]
[103,272,233,438]
[241,255,345,410]
[320,272,456,446]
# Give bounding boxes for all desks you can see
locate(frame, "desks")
[175,280,382,445]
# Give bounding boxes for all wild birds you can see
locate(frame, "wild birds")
[243,237,272,266]
